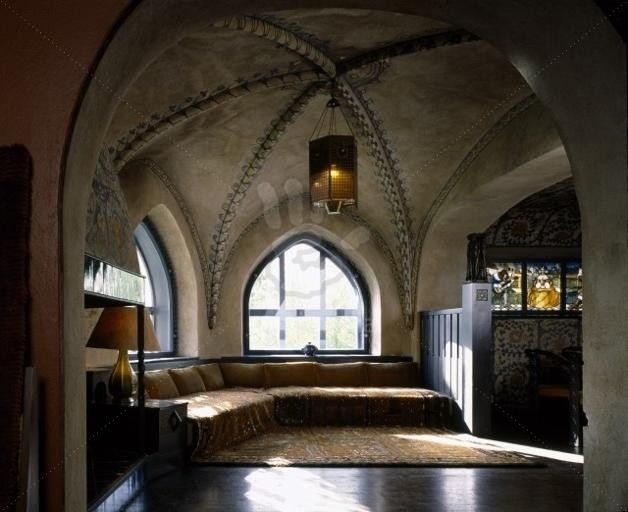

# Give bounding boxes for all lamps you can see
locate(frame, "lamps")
[85,301,162,403]
[307,77,361,218]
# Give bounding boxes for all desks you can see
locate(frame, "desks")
[557,346,584,450]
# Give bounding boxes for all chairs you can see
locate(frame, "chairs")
[520,343,583,442]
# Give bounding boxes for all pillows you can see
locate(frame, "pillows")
[139,357,429,400]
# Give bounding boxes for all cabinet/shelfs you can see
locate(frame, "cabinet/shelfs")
[83,249,154,512]
[89,396,190,480]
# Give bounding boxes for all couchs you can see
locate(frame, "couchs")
[124,353,275,460]
[216,353,462,430]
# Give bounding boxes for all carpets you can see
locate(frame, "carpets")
[190,423,550,470]
